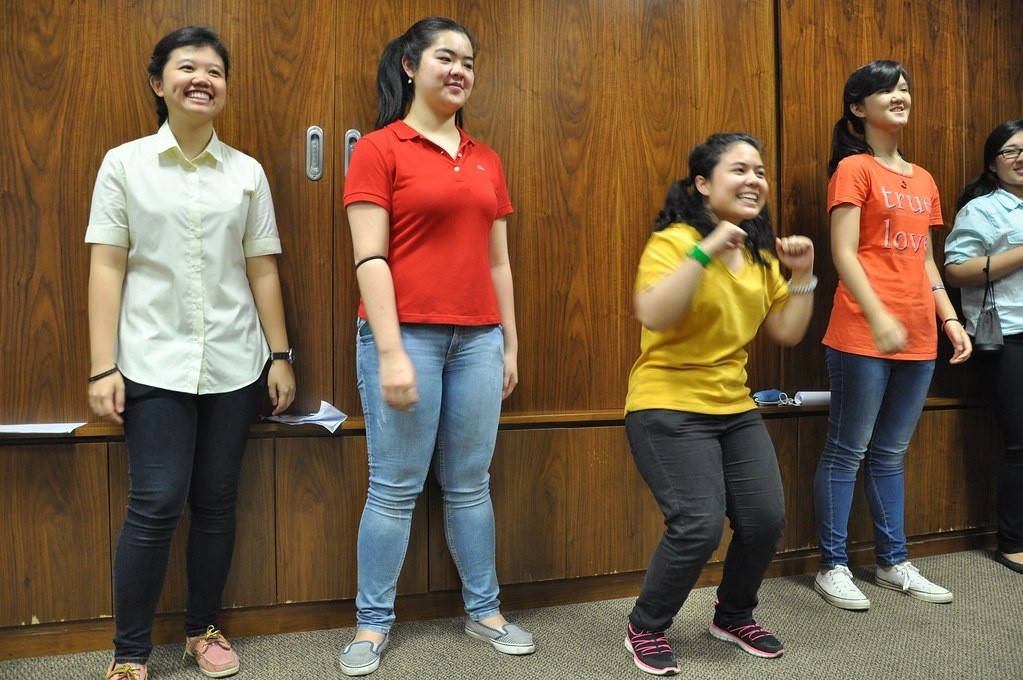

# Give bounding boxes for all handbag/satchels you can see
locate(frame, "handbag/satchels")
[974,255,1004,352]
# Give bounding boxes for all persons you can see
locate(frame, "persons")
[85,24,296,680]
[812,59,972,610]
[623,133,818,675]
[341,15,536,676]
[943,119,1023,575]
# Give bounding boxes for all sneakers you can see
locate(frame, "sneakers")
[624,620,680,676]
[813,564,870,610]
[708,600,784,658]
[182,625,240,677]
[106,657,148,680]
[875,561,954,603]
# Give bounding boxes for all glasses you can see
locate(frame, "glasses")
[997,148,1023,158]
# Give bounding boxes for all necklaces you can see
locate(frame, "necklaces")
[875,152,906,188]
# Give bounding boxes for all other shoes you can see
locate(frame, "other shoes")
[465,617,536,655]
[339,632,389,676]
[995,548,1023,574]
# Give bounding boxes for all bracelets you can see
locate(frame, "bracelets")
[941,318,965,330]
[688,244,713,266]
[87,363,118,382]
[788,277,817,294]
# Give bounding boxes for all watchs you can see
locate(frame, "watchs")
[271,347,295,364]
